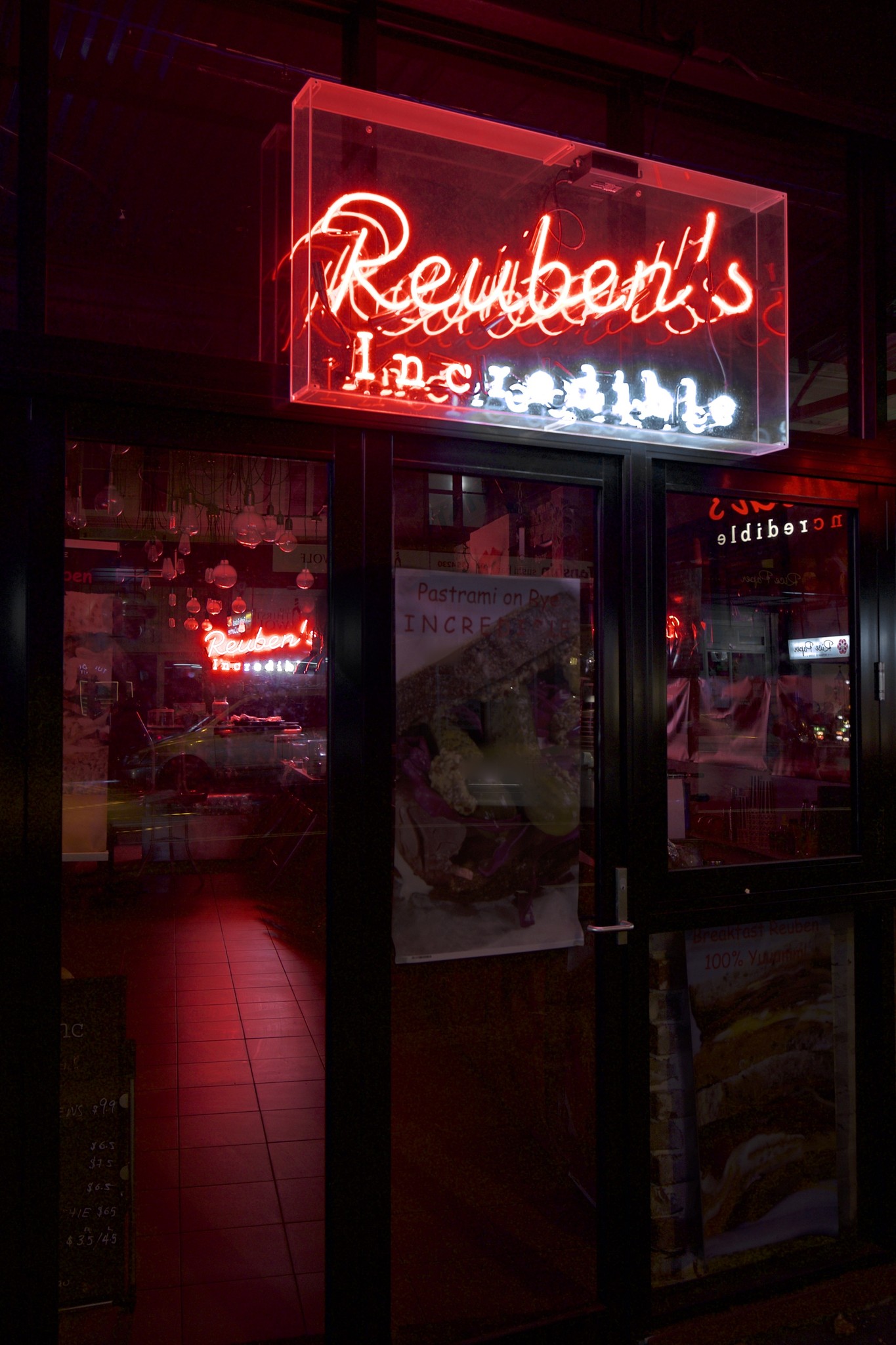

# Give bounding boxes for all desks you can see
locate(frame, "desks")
[131,792,210,895]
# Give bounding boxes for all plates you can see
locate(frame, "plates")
[233,720,285,728]
[281,722,299,725]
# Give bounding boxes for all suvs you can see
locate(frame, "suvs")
[128,685,328,792]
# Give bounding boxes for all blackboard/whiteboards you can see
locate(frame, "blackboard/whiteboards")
[58,971,138,1313]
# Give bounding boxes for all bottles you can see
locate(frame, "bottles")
[767,799,817,858]
[212,696,229,727]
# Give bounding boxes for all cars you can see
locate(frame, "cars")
[98,784,248,867]
